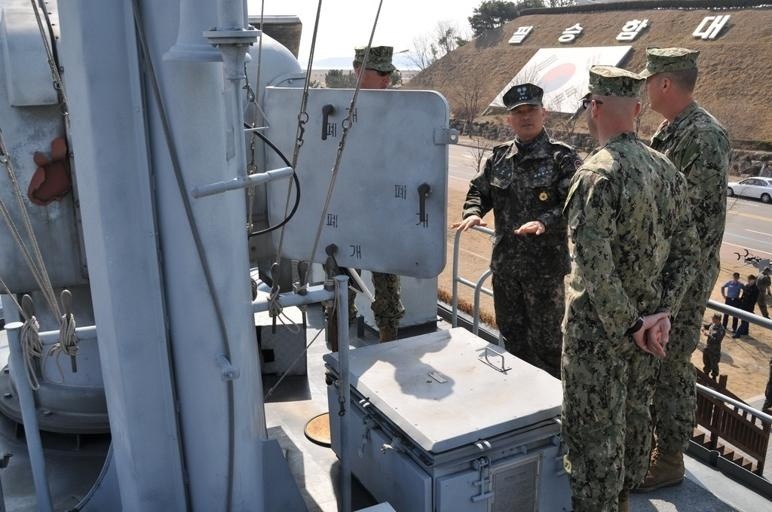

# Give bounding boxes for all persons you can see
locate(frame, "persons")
[721,272,745,332]
[630,47,731,494]
[324,46,406,344]
[732,274,759,338]
[755,266,771,319]
[561,67,700,512]
[451,84,583,381]
[701,312,725,382]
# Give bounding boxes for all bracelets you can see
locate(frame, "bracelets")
[630,318,643,334]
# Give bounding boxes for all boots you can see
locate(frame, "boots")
[631,444,684,493]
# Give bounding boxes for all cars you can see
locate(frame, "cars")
[727,176,771,204]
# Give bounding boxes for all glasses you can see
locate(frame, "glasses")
[581,100,603,109]
[364,68,391,76]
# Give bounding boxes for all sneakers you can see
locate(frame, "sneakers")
[731,329,747,338]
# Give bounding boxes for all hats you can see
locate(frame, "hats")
[502,83,543,110]
[577,64,647,98]
[711,313,722,320]
[354,46,395,73]
[638,45,700,80]
